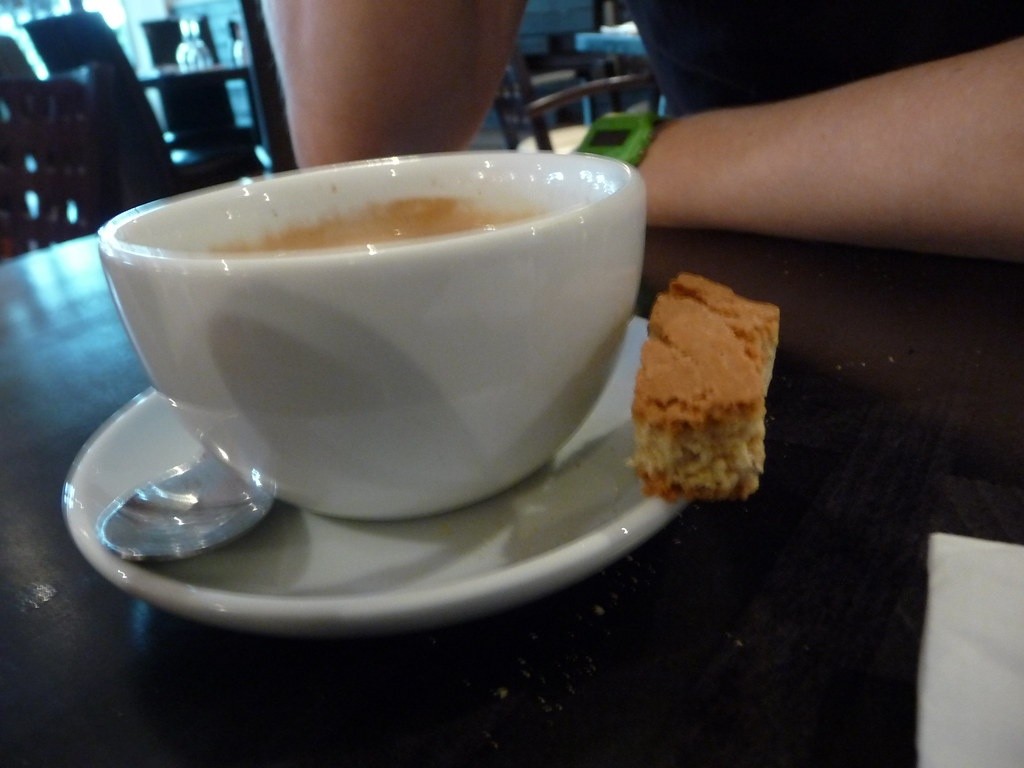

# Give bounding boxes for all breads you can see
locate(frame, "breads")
[629,273,779,502]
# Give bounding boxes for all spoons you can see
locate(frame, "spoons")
[99,455,274,560]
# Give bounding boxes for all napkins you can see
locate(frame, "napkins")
[915,532,1024,768]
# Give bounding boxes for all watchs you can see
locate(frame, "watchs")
[579,110,663,166]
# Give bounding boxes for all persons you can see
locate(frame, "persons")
[259,0,1024,264]
[642,226,1024,468]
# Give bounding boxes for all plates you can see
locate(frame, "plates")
[63,317,694,631]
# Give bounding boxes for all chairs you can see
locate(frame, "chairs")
[0,0,262,263]
[494,58,665,154]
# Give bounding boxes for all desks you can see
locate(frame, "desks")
[0,103,1024,768]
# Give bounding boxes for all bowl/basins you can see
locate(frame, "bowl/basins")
[98,149,645,521]
